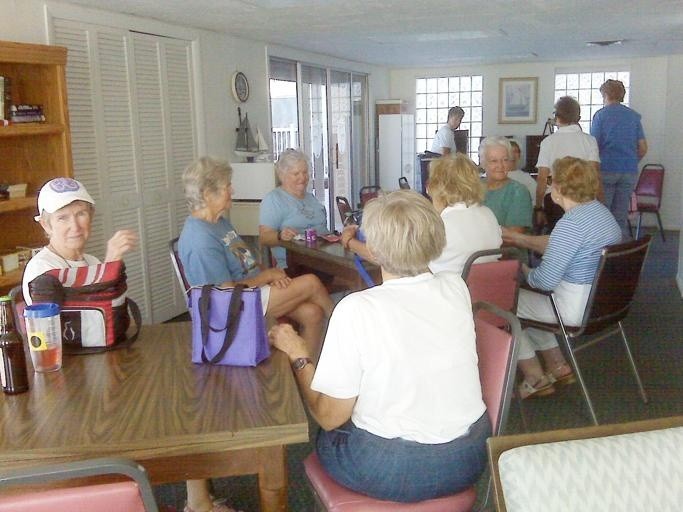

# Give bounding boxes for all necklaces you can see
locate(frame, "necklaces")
[49,244,90,268]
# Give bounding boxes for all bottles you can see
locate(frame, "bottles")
[344,212,357,228]
[0,295,29,396]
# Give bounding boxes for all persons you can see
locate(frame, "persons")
[337,152,502,284]
[475,136,533,287]
[500,156,623,401]
[177,156,335,357]
[505,141,544,222]
[534,98,601,236]
[256,148,332,290]
[267,188,493,503]
[591,79,648,232]
[430,106,464,156]
[22,177,134,308]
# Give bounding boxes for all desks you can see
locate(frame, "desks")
[277,235,382,294]
[1,322,310,509]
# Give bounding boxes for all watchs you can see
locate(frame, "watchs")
[291,358,312,374]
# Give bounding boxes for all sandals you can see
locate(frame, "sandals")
[542,362,578,388]
[513,376,555,401]
[181,495,246,512]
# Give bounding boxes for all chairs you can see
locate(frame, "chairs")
[336,196,354,225]
[1,459,161,511]
[170,237,289,325]
[517,234,650,425]
[462,247,523,316]
[627,164,666,244]
[490,412,682,512]
[304,299,521,510]
[399,177,410,189]
[359,186,383,203]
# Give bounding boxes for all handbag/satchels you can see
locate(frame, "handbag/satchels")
[27,260,142,358]
[352,212,369,264]
[183,283,271,369]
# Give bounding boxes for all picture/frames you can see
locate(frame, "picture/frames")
[496,76,538,127]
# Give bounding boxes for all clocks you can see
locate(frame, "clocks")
[230,70,251,103]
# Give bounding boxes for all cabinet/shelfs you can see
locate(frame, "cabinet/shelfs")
[0,40,76,296]
[525,134,549,174]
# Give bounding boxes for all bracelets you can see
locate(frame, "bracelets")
[344,238,353,249]
[277,231,282,241]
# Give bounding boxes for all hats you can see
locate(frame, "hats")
[33,177,96,223]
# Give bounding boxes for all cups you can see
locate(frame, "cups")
[304,228,318,244]
[20,302,65,375]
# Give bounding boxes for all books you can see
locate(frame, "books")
[0,183,27,199]
[0,76,46,125]
